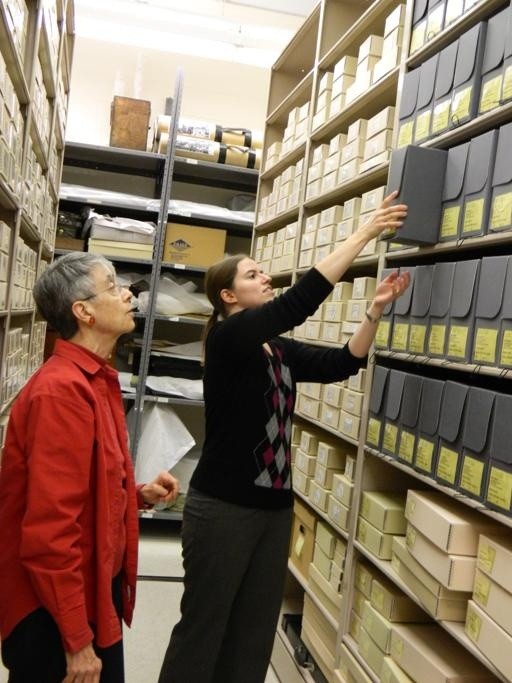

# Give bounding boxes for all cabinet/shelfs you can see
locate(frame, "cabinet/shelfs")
[1,0,79,452]
[44,71,260,522]
[251,1,509,682]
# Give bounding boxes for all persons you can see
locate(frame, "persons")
[158,190,410,683]
[0,251,179,683]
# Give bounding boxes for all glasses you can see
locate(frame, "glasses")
[82,281,122,302]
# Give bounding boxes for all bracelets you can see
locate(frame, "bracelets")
[366,311,382,323]
